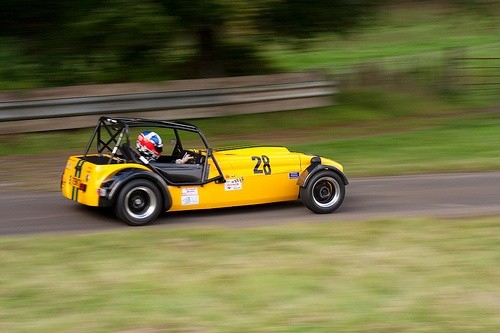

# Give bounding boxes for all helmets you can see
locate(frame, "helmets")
[136,130,163,160]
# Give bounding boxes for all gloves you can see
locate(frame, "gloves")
[181,152,194,164]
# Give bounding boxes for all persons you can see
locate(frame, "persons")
[136,131,194,164]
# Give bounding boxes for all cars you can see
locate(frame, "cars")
[60,114,351,226]
